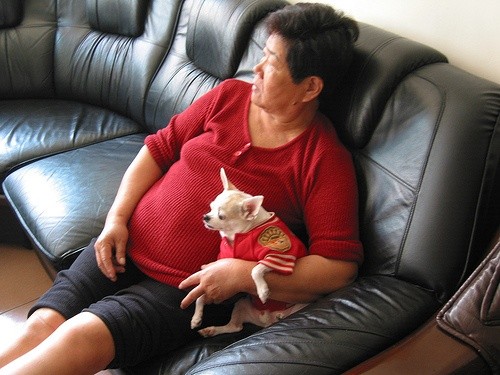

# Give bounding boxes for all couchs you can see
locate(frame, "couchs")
[0,0,500,375]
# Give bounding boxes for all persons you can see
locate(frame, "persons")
[0,2,365,375]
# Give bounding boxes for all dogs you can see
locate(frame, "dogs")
[192,167,308,337]
